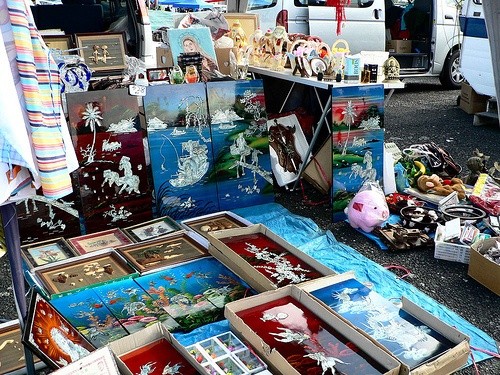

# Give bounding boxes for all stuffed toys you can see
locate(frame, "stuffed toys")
[417,171,467,200]
[344,189,389,232]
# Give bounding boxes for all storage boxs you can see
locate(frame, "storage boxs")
[108,322,210,375]
[459,82,490,113]
[301,136,333,193]
[299,271,472,375]
[434,227,492,264]
[207,222,338,292]
[468,236,500,297]
[386,39,411,53]
[225,284,401,375]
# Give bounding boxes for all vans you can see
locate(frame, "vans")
[245,0,500,126]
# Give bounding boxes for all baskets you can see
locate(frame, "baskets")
[434,224,472,264]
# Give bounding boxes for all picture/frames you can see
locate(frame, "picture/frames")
[0,215,221,375]
[75,31,129,76]
[222,13,259,46]
[41,35,74,56]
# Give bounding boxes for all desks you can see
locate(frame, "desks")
[240,65,403,224]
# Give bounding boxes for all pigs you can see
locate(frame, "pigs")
[344,189,391,232]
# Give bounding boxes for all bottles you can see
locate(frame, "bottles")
[360,64,370,84]
